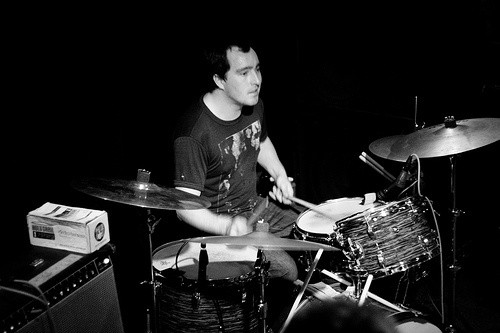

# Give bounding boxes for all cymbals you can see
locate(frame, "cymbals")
[81,179,211,210]
[179,233,342,251]
[391,117,500,159]
[369,135,416,162]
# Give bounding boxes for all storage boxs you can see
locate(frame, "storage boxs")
[26,201,111,255]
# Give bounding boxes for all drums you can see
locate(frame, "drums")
[152,240,271,333]
[334,195,441,284]
[293,197,388,280]
[295,283,446,333]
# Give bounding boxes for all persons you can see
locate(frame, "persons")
[171,36,302,283]
[285,299,394,333]
